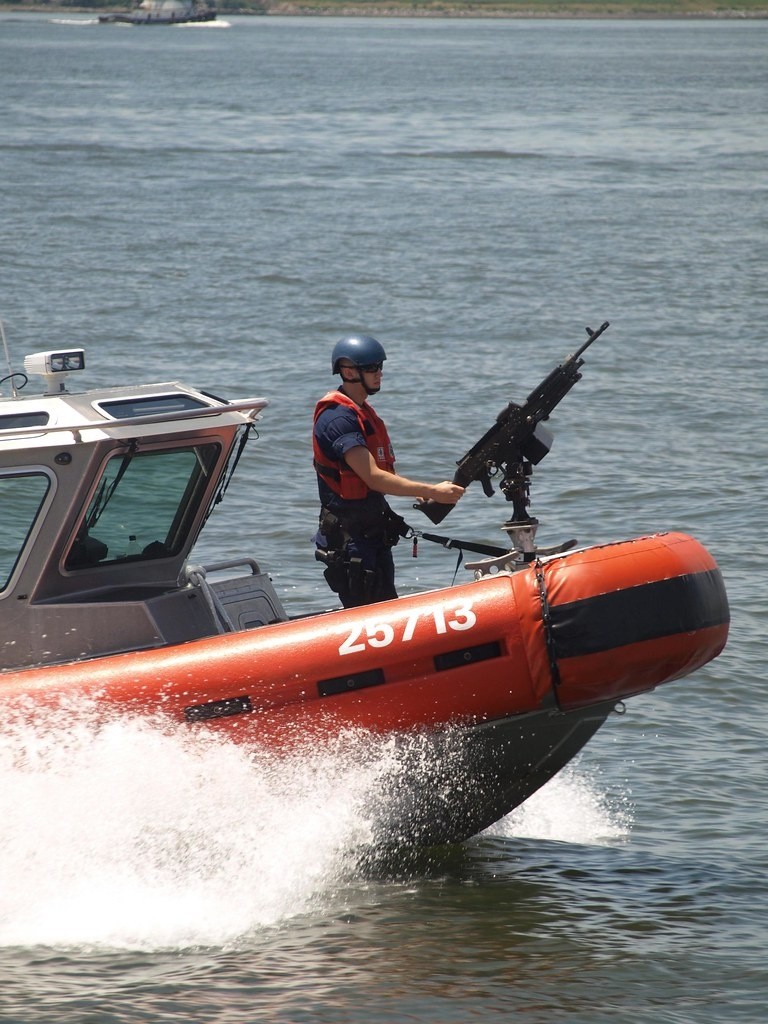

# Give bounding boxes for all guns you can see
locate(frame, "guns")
[413,322,612,526]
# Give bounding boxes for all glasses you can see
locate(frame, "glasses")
[341,362,383,372]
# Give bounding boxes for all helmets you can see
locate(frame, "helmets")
[332,335,387,374]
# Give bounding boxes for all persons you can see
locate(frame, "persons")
[311,334,466,610]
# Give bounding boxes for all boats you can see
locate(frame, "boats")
[1,345,731,899]
[97,1,220,25]
